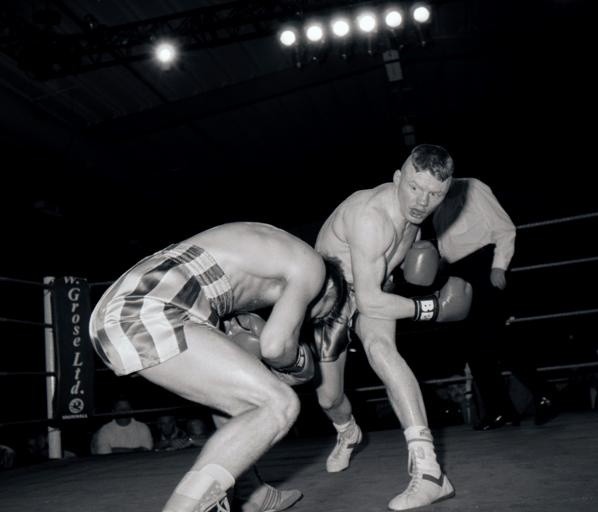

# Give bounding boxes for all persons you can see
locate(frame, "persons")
[86,218,349,512]
[0,398,215,470]
[437,374,467,426]
[311,142,477,511]
[432,175,567,431]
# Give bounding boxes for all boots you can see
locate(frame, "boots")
[389,425,455,510]
[326,415,362,473]
[163,463,235,512]
[235,465,302,512]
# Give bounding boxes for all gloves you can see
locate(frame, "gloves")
[271,342,316,386]
[414,276,472,323]
[224,314,265,361]
[403,241,438,287]
